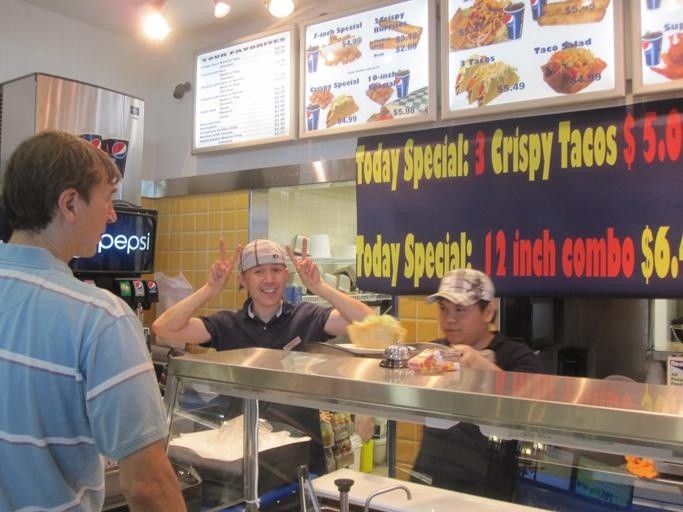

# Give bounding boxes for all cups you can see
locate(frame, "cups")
[503,1,544,40]
[641,34,664,66]
[647,0,662,10]
[305,50,320,73]
[394,72,410,98]
[306,108,319,130]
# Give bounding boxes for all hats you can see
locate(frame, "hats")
[426,268,495,307]
[238,239,286,291]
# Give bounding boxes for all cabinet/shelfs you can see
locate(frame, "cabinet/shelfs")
[283,252,393,304]
[153,347,682,509]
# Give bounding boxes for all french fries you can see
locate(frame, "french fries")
[369,20,422,50]
[538,0,610,26]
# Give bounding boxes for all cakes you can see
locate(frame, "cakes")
[345,314,406,349]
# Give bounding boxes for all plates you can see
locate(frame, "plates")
[338,341,415,354]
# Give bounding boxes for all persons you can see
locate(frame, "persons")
[402,272,540,505]
[0,126,197,512]
[147,236,375,480]
